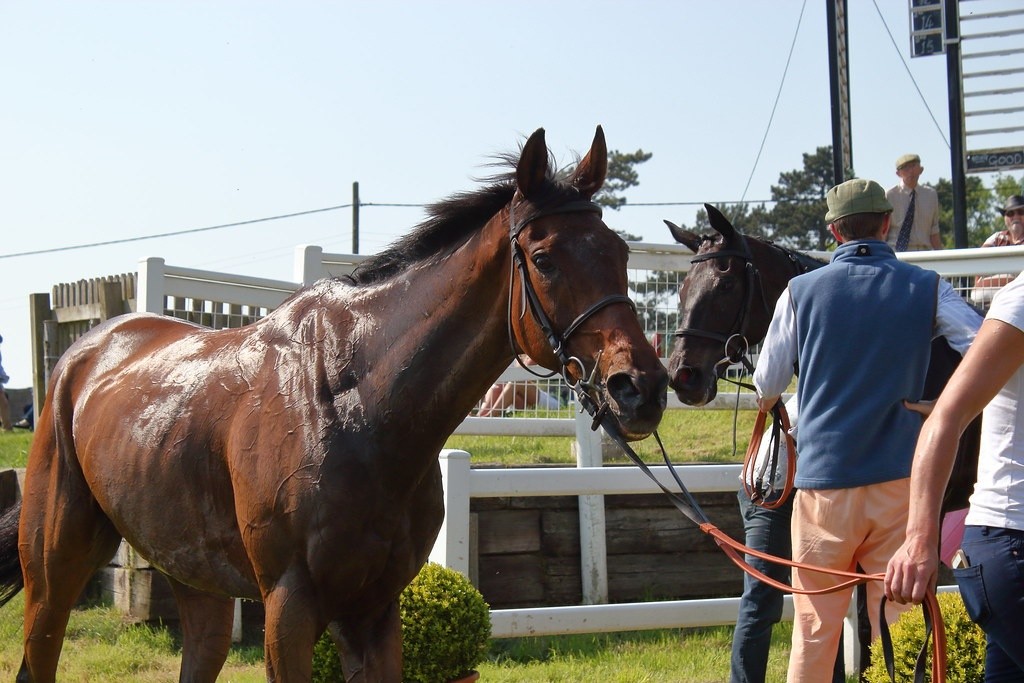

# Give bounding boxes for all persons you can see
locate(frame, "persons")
[884,272,1024,683]
[885,154,944,252]
[0,334,10,425]
[471,355,560,415]
[753,180,983,683]
[971,196,1024,301]
[12,388,34,431]
[729,392,873,683]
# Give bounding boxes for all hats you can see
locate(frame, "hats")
[897,155,921,169]
[824,179,893,224]
[996,195,1024,216]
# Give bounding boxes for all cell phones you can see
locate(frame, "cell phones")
[950,549,969,569]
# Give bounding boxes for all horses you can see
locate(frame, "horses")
[0,124,989,683]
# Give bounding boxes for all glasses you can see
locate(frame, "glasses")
[1004,209,1024,217]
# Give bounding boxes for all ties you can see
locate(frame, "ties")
[894,189,914,252]
